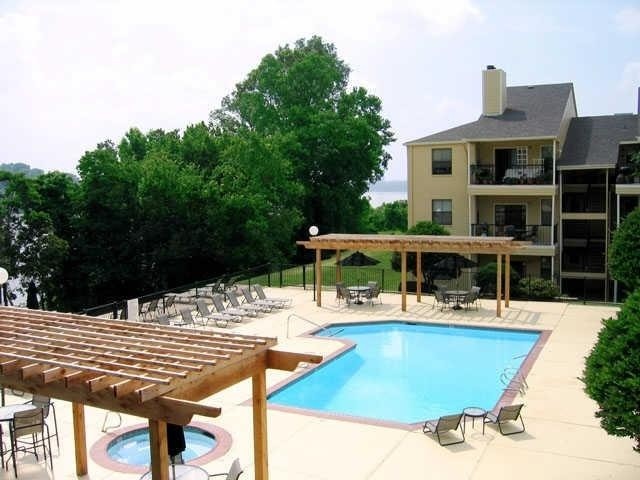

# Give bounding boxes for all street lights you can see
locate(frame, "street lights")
[309,226,319,301]
[0,268,8,305]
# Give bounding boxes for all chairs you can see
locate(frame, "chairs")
[423,402,525,447]
[111,275,289,328]
[1,389,60,478]
[431,279,481,313]
[336,280,383,309]
[210,457,243,480]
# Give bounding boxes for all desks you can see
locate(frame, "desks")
[139,462,209,480]
[503,167,546,184]
